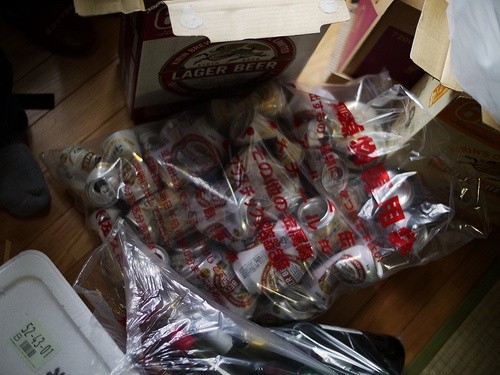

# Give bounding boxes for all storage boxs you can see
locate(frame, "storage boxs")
[74,0,350,123]
[295,0,435,110]
[0,249,140,375]
[385,75,499,213]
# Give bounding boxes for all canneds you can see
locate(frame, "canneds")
[59,86,450,321]
